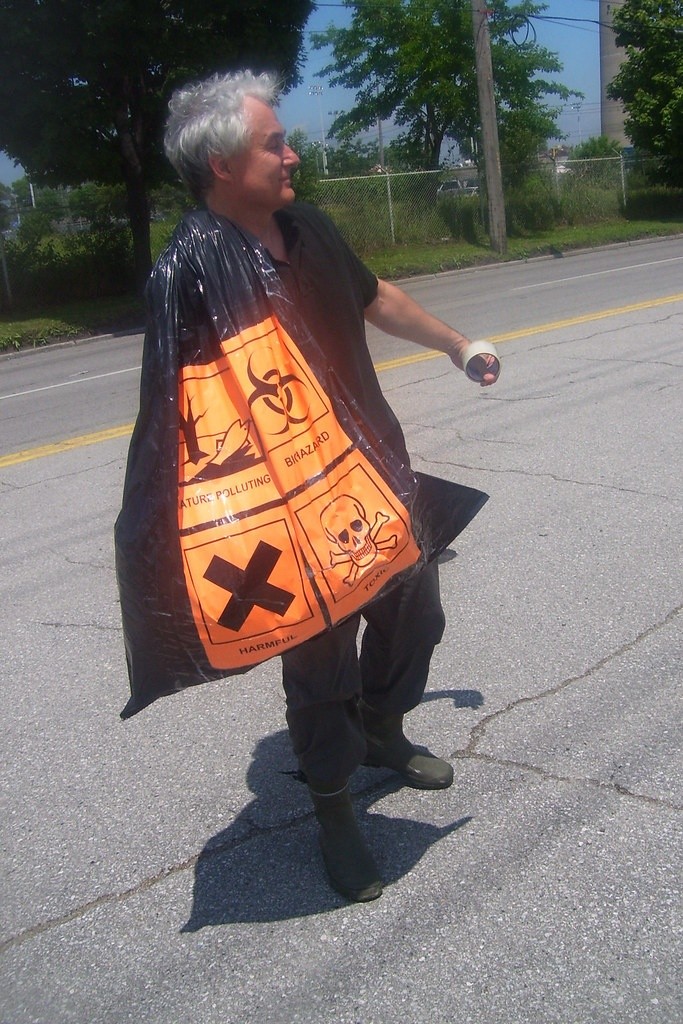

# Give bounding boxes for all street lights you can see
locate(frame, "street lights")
[306,84,324,150]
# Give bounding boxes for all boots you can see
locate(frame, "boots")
[357,697,453,789]
[309,776,382,903]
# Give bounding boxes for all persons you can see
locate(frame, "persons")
[146,68,497,902]
